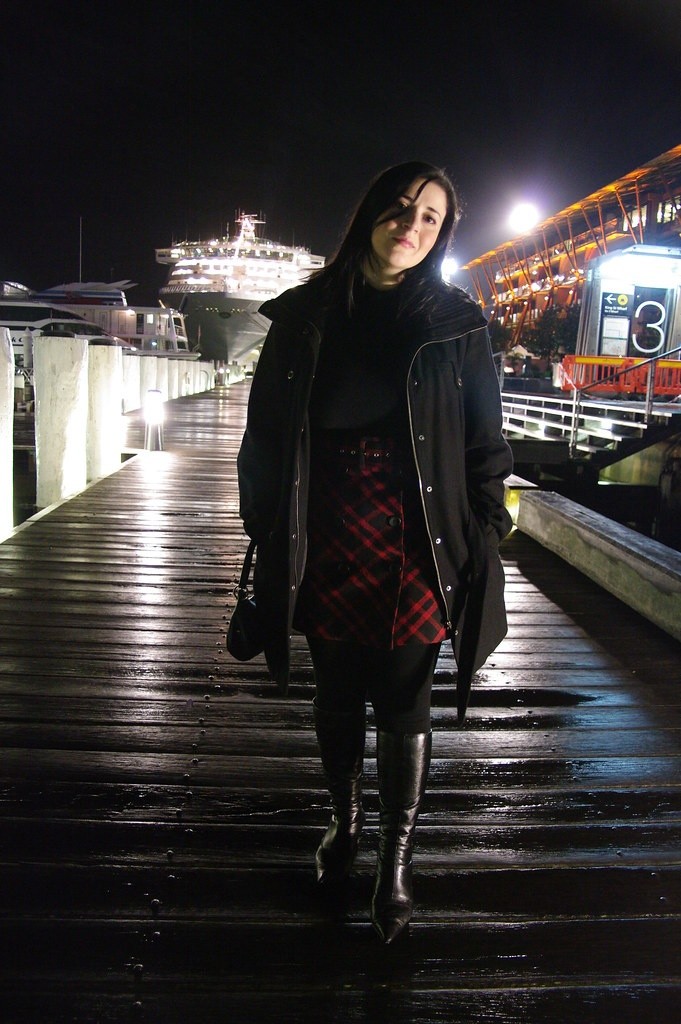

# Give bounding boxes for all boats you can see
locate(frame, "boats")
[156,206,327,370]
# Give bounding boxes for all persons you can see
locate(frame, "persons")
[238,162,513,945]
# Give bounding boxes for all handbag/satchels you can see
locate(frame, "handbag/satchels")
[227,586,265,662]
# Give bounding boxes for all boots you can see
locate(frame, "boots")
[312,696,368,883]
[372,727,433,944]
